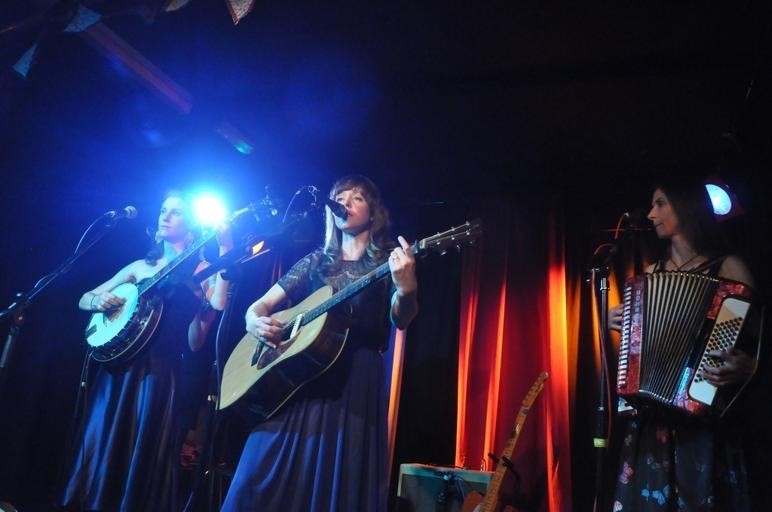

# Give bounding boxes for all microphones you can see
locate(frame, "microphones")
[106,205,137,219]
[625,206,644,223]
[309,186,348,220]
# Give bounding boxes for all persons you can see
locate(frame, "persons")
[187,299,216,353]
[607,179,764,511]
[64,187,238,512]
[218,174,419,512]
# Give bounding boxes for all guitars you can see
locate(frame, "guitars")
[461,372,549,512]
[215,214,485,428]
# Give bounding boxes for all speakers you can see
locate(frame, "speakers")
[395,463,494,512]
